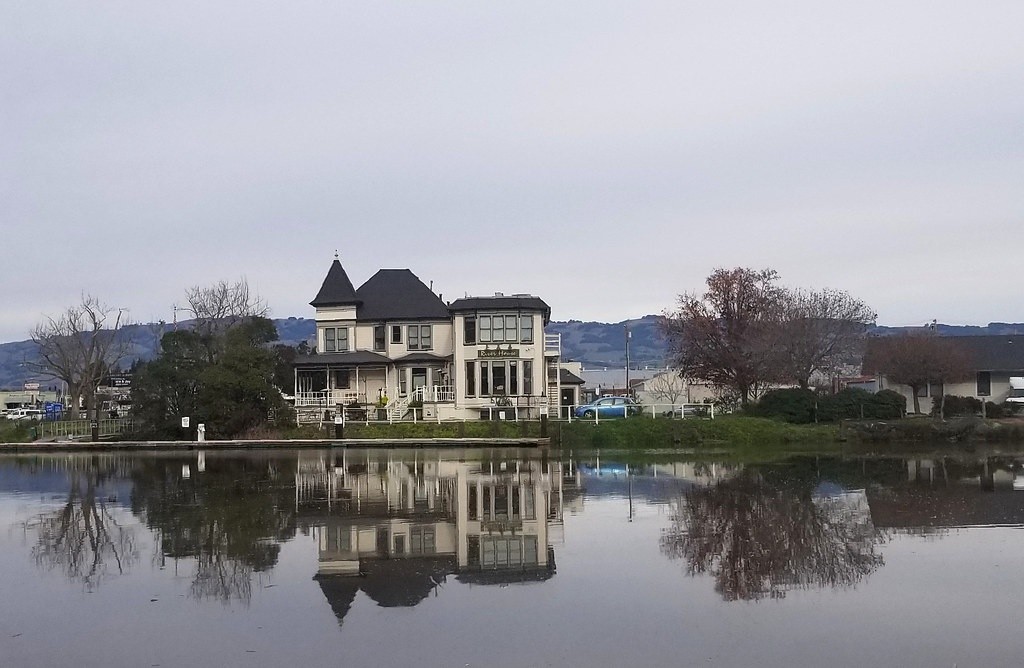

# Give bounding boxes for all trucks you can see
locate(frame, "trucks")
[1005,377,1024,407]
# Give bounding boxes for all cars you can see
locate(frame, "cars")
[6,411,19,419]
[582,463,643,478]
[575,397,642,419]
[19,410,47,421]
[662,404,711,418]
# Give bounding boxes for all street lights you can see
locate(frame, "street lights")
[20,362,24,408]
[623,325,629,397]
[628,469,632,522]
